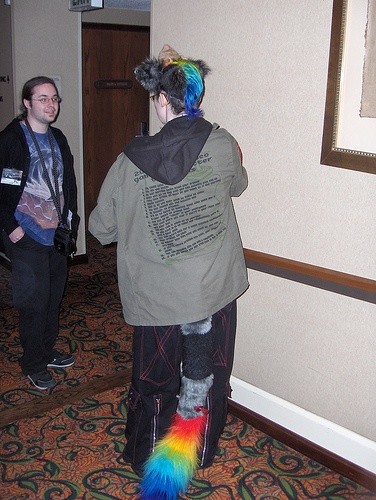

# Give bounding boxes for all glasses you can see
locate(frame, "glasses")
[23,96,61,104]
[149,91,168,101]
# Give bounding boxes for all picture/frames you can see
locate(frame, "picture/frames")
[321,0,376,175]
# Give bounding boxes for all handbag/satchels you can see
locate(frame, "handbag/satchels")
[53,227,76,257]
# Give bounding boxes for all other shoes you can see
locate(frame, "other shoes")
[25,369,56,391]
[47,349,75,367]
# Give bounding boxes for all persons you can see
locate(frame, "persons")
[0,76,81,389]
[89,45,250,500]
